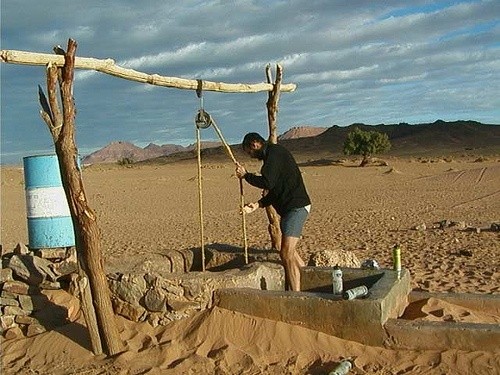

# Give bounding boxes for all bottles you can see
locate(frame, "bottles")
[332,264,343,295]
[329,359,354,375]
[342,285,368,301]
[393,243,401,271]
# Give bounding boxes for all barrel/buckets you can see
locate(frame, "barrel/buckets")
[23,154,81,248]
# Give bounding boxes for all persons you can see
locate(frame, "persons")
[236,132,311,292]
